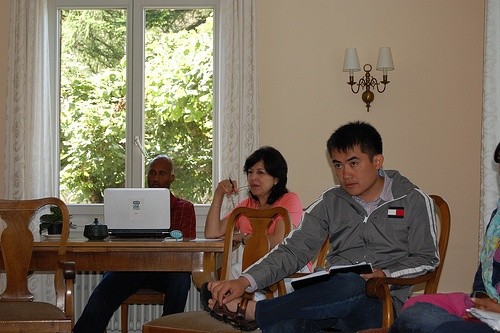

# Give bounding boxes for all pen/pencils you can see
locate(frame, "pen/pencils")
[229,177,234,188]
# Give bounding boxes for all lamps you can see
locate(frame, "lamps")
[343,46,395,112]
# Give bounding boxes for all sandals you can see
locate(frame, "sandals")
[199,281,259,332]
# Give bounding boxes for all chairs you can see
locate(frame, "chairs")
[279,194,451,333]
[120,290,166,333]
[141,206,293,333]
[0,197,73,333]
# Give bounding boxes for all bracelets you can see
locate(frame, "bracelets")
[211,202,221,208]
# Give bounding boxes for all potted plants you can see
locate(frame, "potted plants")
[38,206,74,239]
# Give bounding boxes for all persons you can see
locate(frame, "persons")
[389,141,500,333]
[206,147,313,302]
[201,122,440,333]
[72,155,196,333]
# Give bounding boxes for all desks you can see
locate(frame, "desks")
[0,235,226,333]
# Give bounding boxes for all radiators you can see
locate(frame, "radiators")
[75,272,206,331]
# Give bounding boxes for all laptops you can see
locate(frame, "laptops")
[104,188,171,238]
